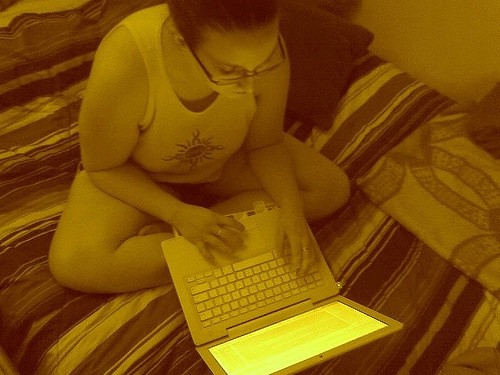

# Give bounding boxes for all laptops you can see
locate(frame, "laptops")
[158,204,405,375]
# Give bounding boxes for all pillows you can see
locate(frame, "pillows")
[278,0,375,130]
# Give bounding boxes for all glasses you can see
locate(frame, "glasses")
[184,34,287,86]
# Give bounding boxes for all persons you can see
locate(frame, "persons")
[48,0,352,294]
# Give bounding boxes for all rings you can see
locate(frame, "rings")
[214,229,223,236]
[302,247,311,252]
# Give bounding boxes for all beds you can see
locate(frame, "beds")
[0,0,500,375]
